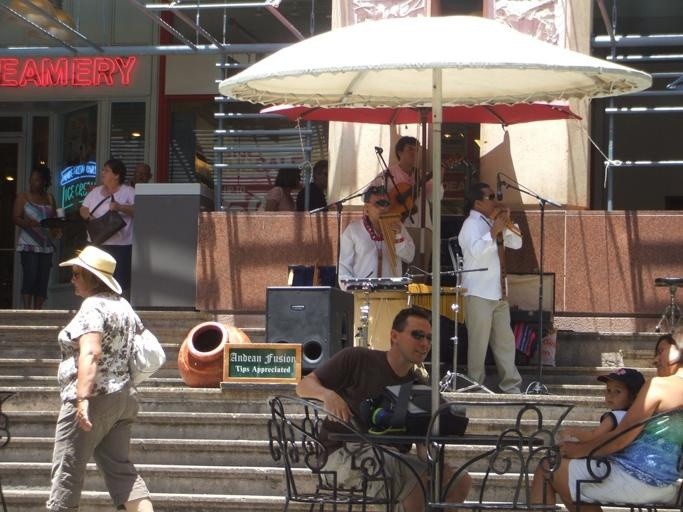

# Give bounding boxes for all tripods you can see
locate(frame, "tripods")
[504,182,562,398]
[405,267,497,394]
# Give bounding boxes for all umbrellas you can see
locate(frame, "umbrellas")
[218,15,653,438]
[256,98,586,283]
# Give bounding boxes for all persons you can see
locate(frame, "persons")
[260,164,299,213]
[289,157,337,287]
[524,330,683,512]
[78,156,137,297]
[455,182,526,395]
[550,364,646,462]
[337,183,418,293]
[129,163,154,189]
[44,237,156,512]
[290,305,476,511]
[8,160,68,310]
[363,136,447,232]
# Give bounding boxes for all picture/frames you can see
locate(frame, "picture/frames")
[224,342,303,387]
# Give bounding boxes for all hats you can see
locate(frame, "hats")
[597,369,645,390]
[59,246,123,295]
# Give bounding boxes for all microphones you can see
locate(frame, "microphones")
[375,146,383,153]
[496,174,505,202]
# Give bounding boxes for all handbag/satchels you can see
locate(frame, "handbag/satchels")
[376,383,468,437]
[257,186,284,211]
[129,328,167,387]
[86,210,127,245]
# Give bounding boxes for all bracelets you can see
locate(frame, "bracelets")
[77,396,92,402]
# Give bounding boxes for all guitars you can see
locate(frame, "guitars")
[389,151,467,222]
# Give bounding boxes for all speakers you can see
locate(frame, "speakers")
[266,286,354,376]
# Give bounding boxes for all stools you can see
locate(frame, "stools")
[655,276,683,334]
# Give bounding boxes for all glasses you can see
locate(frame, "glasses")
[404,329,432,342]
[483,194,495,201]
[71,270,80,280]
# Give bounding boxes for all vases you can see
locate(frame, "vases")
[178,321,253,388]
